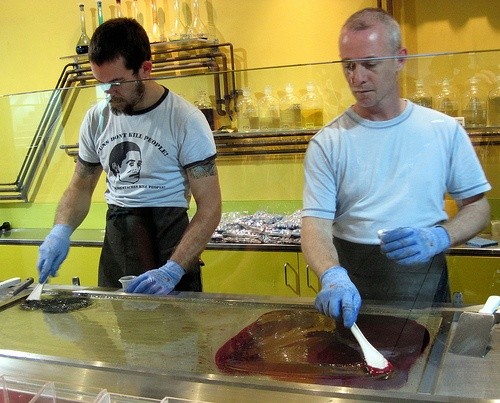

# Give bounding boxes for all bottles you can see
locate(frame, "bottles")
[461,78,485,127]
[301,85,324,130]
[76,4,90,54]
[486,77,500,127]
[185,0,209,40]
[279,86,304,129]
[168,0,189,40]
[236,90,259,131]
[96,0,147,43]
[409,84,432,110]
[193,92,214,131]
[258,88,280,131]
[435,82,459,120]
[148,1,167,43]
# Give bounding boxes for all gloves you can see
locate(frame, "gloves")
[37,224,74,284]
[380,225,451,266]
[124,260,185,295]
[315,266,362,329]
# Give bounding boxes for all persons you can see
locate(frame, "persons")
[301,9,492,328]
[38,18,222,295]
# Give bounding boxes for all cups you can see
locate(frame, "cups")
[378,228,395,255]
[118,275,136,292]
[491,220,500,236]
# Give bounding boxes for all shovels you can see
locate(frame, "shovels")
[26,277,48,301]
[350,321,390,368]
[447,295,500,357]
